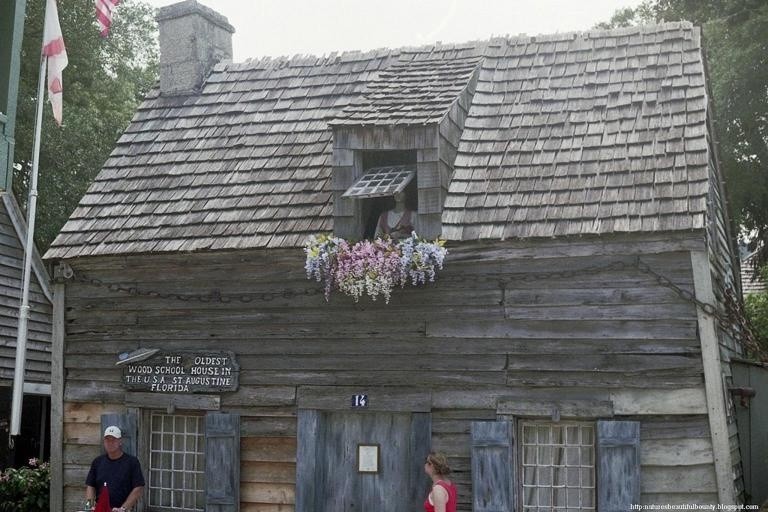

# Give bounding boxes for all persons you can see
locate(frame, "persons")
[85,425,145,512]
[374,186,418,240]
[423,451,457,512]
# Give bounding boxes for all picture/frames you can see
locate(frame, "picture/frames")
[356,442,381,474]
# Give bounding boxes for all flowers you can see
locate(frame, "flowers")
[303,231,450,305]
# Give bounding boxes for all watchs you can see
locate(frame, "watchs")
[120,506,127,511]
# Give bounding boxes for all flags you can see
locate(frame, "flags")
[94,0,114,31]
[41,0,68,125]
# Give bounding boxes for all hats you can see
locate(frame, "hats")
[104,425,122,439]
